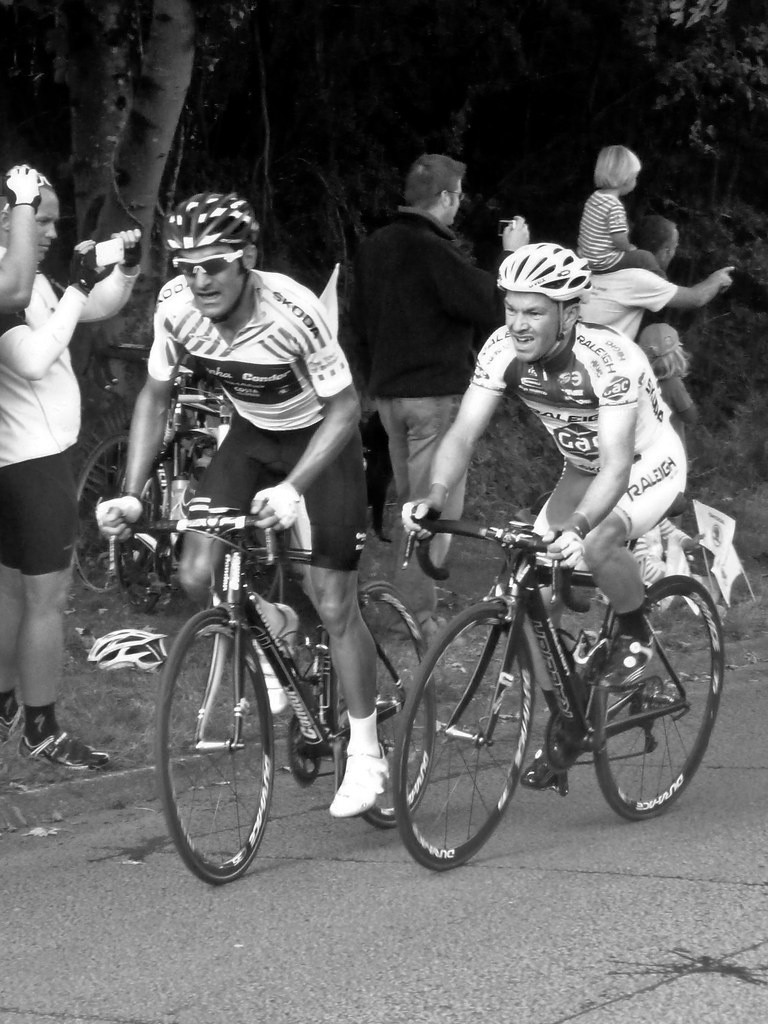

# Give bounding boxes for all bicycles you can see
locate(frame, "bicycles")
[390,508,723,871]
[114,498,437,884]
[70,332,228,613]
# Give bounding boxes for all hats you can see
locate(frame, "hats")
[640,323,684,356]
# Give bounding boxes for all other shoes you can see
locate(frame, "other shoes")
[391,616,464,650]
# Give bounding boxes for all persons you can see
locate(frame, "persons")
[319,154,530,638]
[576,144,668,280]
[95,189,391,818]
[402,243,686,788]
[0,163,143,772]
[578,216,735,342]
[637,324,700,427]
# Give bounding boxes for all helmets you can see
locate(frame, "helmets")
[86,629,169,675]
[160,193,260,250]
[496,243,592,304]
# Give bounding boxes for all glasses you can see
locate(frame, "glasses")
[172,242,258,277]
[4,175,52,211]
[436,190,466,201]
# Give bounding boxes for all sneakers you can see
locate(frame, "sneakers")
[18,728,110,771]
[252,603,299,714]
[600,631,656,686]
[0,705,25,746]
[521,740,581,788]
[330,742,390,818]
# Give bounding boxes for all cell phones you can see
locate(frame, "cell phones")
[95,237,125,267]
[497,219,516,236]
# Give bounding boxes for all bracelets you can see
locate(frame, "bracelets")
[123,492,141,500]
